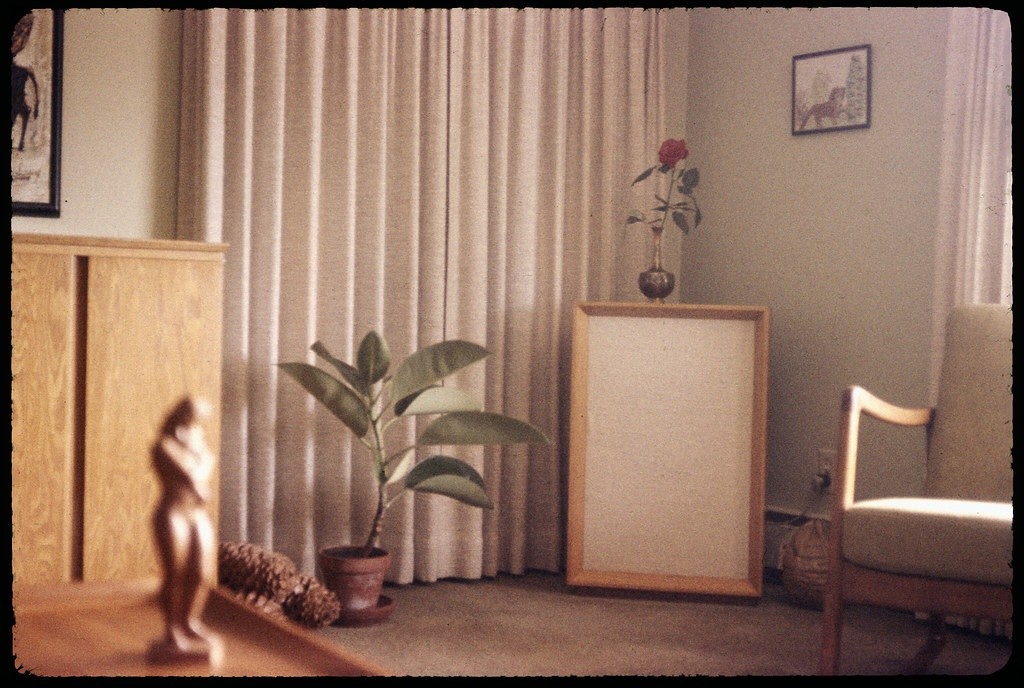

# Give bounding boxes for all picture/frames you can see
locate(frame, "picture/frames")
[791,41,873,137]
[10,7,66,219]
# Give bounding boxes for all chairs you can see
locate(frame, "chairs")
[815,301,1013,677]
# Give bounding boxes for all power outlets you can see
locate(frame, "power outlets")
[817,447,838,493]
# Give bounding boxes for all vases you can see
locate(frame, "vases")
[637,225,676,303]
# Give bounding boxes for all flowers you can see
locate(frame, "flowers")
[625,136,704,237]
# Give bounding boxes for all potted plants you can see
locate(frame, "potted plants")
[273,331,550,627]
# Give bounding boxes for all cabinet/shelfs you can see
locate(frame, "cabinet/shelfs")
[13,229,230,584]
[567,299,771,599]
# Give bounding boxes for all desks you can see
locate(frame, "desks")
[10,581,383,675]
